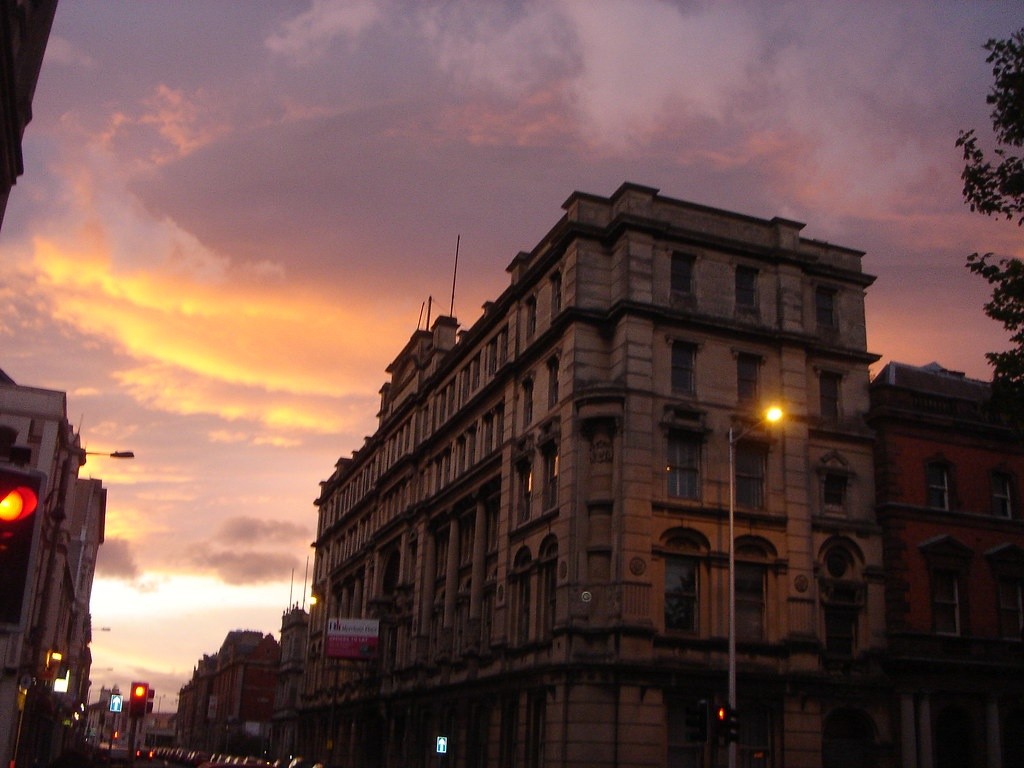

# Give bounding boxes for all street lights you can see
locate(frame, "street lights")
[728,407,784,768]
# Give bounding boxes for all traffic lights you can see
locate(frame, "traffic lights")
[0,467,46,638]
[130,681,155,720]
